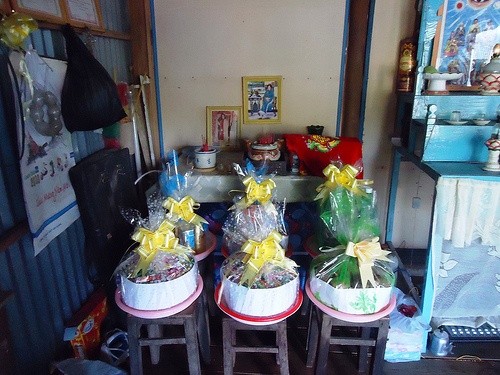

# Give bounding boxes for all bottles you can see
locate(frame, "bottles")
[291,154,300,174]
[476,57,500,95]
[470,58,487,86]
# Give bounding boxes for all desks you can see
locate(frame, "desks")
[162,148,324,202]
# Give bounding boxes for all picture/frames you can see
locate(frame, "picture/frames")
[243,76,282,124]
[206,106,243,151]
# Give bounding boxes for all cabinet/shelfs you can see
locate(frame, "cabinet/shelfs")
[385,93,500,328]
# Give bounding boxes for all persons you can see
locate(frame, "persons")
[219,115,230,141]
[262,84,275,113]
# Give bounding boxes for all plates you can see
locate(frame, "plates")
[115,274,204,319]
[473,120,491,125]
[303,233,319,258]
[192,229,218,261]
[221,242,294,257]
[214,282,304,326]
[444,119,468,125]
[305,278,396,323]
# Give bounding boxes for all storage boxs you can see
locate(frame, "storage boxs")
[64,287,112,359]
[384,323,433,363]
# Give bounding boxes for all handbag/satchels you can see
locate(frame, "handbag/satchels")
[385,287,430,363]
[61,23,127,133]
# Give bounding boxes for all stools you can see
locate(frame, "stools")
[301,254,388,375]
[219,313,290,375]
[126,252,216,375]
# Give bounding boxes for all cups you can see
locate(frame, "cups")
[194,147,216,168]
[450,110,461,122]
[307,125,324,135]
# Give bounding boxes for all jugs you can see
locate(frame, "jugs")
[428,327,454,357]
